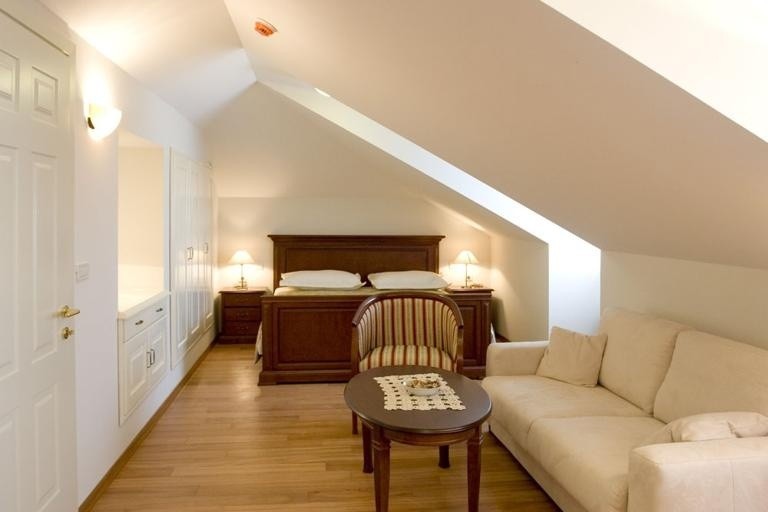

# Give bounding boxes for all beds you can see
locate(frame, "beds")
[253,234,494,385]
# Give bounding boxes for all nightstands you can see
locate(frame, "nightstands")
[444,285,496,292]
[218,288,266,344]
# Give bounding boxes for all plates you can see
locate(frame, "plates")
[402,378,445,396]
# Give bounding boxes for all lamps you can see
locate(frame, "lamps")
[227,249,255,289]
[454,249,480,288]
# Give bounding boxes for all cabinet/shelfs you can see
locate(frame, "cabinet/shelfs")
[170,147,215,367]
[118,291,170,426]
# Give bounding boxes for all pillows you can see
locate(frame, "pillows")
[278,270,367,290]
[598,307,687,413]
[535,326,608,388]
[642,409,768,443]
[365,270,449,290]
[651,329,768,423]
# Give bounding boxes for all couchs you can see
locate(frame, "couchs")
[483,338,768,512]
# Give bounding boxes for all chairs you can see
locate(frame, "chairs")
[349,290,464,433]
